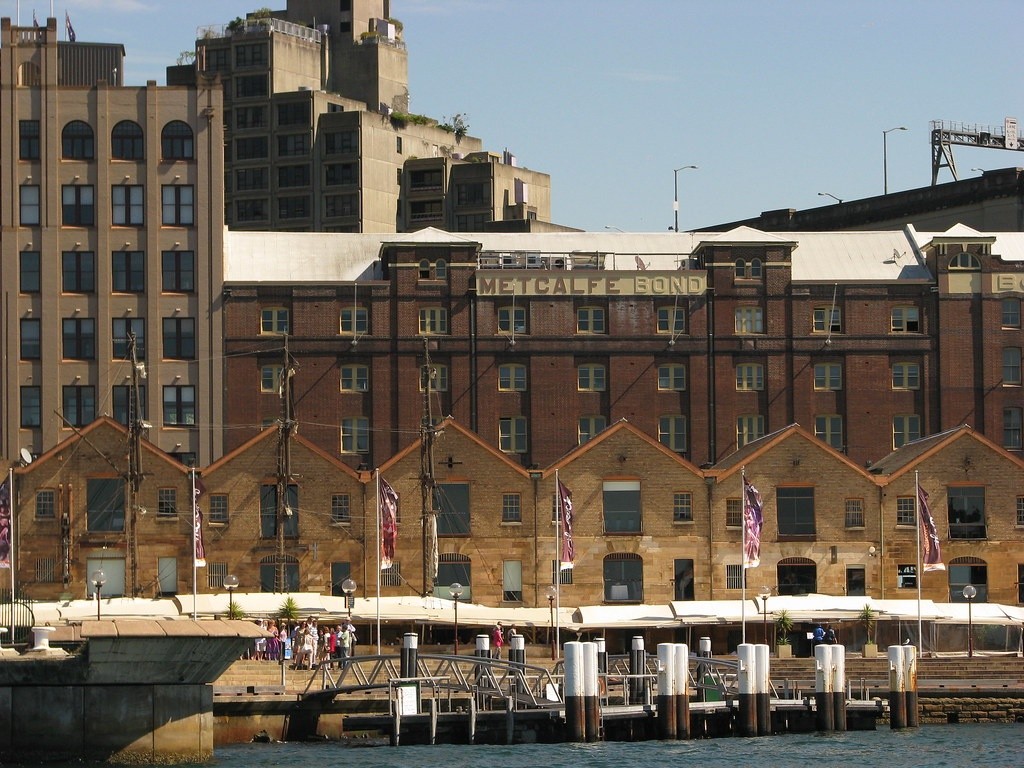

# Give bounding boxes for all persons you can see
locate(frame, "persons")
[453,622,517,658]
[814,624,838,646]
[239,619,405,672]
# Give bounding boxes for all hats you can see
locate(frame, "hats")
[828,625,833,628]
[817,624,821,627]
[345,617,351,621]
[280,623,286,627]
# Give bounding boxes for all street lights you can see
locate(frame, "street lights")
[881,126,909,194]
[962,585,976,661]
[90,569,107,621]
[672,165,699,232]
[758,586,772,644]
[818,192,843,204]
[223,574,239,622]
[545,586,557,661]
[449,583,465,656]
[342,578,357,656]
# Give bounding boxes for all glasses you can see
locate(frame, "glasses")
[281,626,284,627]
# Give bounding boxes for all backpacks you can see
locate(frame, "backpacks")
[825,631,833,642]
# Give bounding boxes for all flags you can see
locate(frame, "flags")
[33,13,43,38]
[66,13,77,42]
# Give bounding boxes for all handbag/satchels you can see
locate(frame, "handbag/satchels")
[509,632,513,642]
